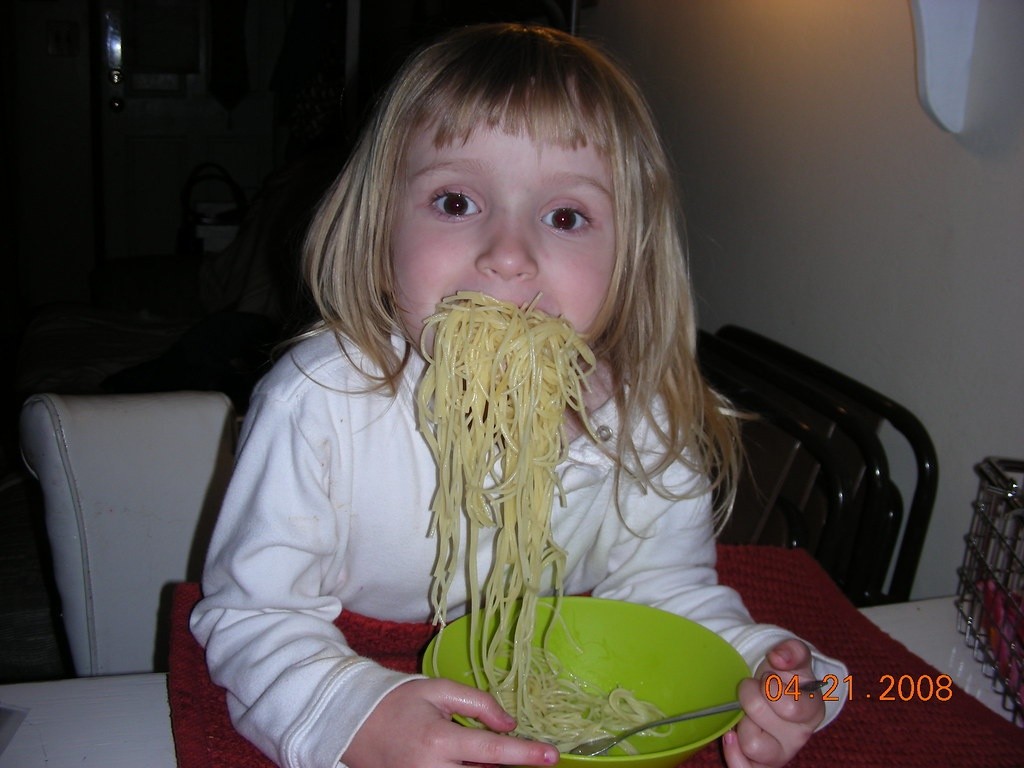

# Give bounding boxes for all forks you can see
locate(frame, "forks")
[570,680,829,754]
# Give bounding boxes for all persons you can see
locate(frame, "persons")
[189,22,850,768]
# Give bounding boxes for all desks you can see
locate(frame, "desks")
[0,596,1023,768]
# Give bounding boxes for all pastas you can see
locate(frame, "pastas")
[413,291,651,755]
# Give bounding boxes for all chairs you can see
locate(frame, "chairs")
[695,324,942,611]
[17,392,247,678]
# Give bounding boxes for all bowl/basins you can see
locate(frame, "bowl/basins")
[421,595,752,768]
